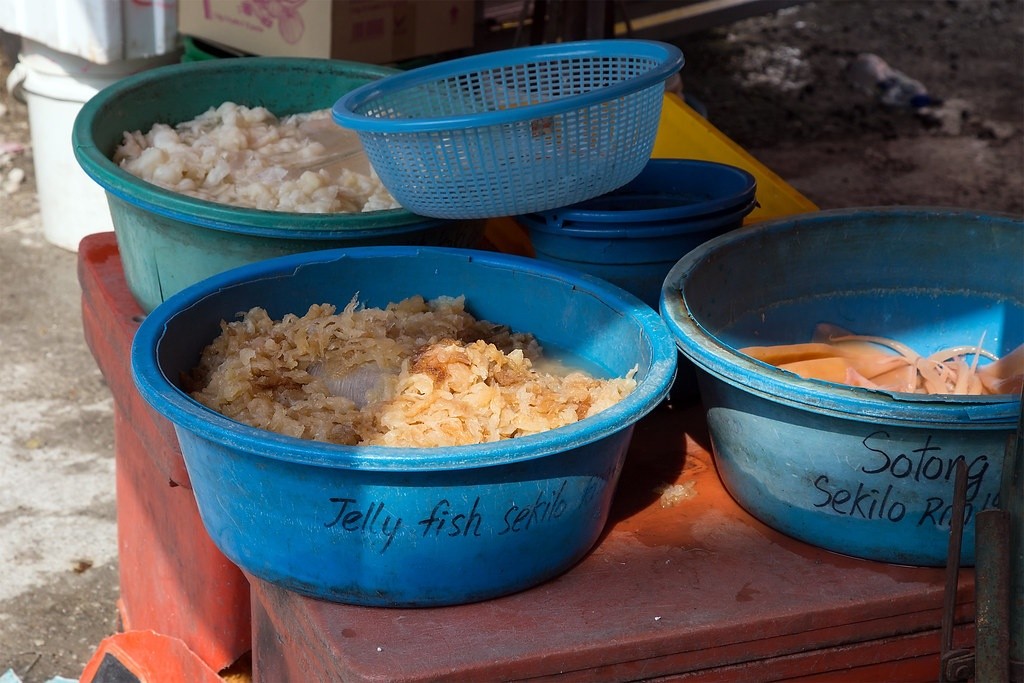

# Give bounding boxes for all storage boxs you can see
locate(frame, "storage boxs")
[2,0,1023,683]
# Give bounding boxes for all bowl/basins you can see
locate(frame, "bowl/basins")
[661,197,1023,568]
[72,54,468,324]
[125,240,680,614]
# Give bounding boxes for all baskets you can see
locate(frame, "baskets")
[330,36,686,220]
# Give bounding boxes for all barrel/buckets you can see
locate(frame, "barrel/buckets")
[4,35,183,252]
[510,157,760,410]
